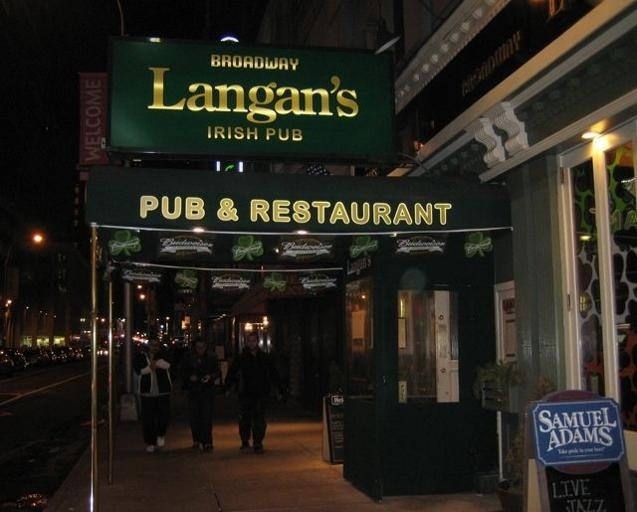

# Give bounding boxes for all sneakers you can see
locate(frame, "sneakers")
[239,442,263,454]
[144,435,166,453]
[192,440,214,451]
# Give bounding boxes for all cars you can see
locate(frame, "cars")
[0,337,141,378]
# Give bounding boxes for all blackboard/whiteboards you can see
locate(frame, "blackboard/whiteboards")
[324,396,346,464]
[536,456,636,511]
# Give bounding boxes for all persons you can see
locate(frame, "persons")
[223,331,281,454]
[179,335,223,452]
[131,336,174,452]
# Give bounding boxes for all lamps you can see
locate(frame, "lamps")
[373,0,401,56]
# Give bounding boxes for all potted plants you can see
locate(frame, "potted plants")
[475,359,557,512]
